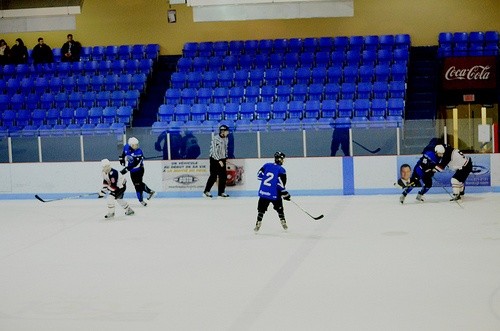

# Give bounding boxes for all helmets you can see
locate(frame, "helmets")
[101,159,109,166]
[274,152,285,159]
[220,124,229,130]
[434,145,445,153]
[128,137,139,149]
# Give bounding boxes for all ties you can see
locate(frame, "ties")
[406,182,409,186]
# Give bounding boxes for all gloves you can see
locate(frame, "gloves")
[119,156,125,166]
[219,160,224,167]
[98,190,106,198]
[120,167,129,175]
[281,191,291,201]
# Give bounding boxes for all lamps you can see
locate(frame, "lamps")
[166,4,177,23]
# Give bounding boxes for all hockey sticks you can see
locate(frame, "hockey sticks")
[352,139,380,153]
[35,193,99,202]
[394,174,425,190]
[142,155,163,159]
[290,198,324,220]
[447,165,481,173]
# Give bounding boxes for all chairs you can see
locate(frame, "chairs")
[437,30,498,57]
[0,33,411,138]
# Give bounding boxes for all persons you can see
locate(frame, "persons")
[31,37,53,64]
[60,35,83,60]
[254,152,291,230]
[0,38,29,65]
[432,145,472,201]
[203,125,230,198]
[119,137,155,207]
[395,164,412,190]
[227,129,237,160]
[96,158,134,218]
[155,126,200,161]
[330,116,353,158]
[399,133,450,203]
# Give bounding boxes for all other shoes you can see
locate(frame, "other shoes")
[218,193,229,198]
[141,203,147,208]
[104,213,115,218]
[282,222,289,230]
[125,208,135,215]
[400,191,407,204]
[254,221,261,231]
[203,190,213,198]
[449,194,461,201]
[147,191,155,200]
[460,190,465,195]
[416,191,424,202]
